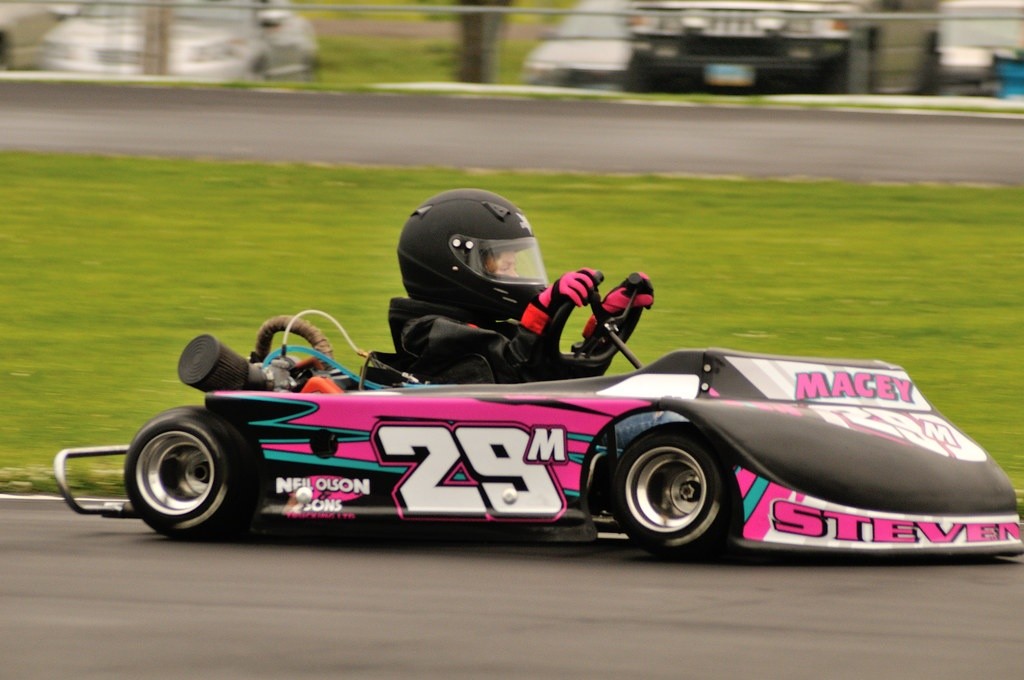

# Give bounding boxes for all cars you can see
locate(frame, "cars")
[520,0,628,90]
[37,1,320,82]
[923,1,1024,99]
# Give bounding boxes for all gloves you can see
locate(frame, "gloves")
[582,273,655,338]
[538,266,604,309]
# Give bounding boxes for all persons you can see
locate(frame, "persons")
[388,187,655,385]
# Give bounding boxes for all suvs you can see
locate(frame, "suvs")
[627,1,853,97]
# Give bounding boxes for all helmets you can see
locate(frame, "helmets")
[399,188,547,323]
